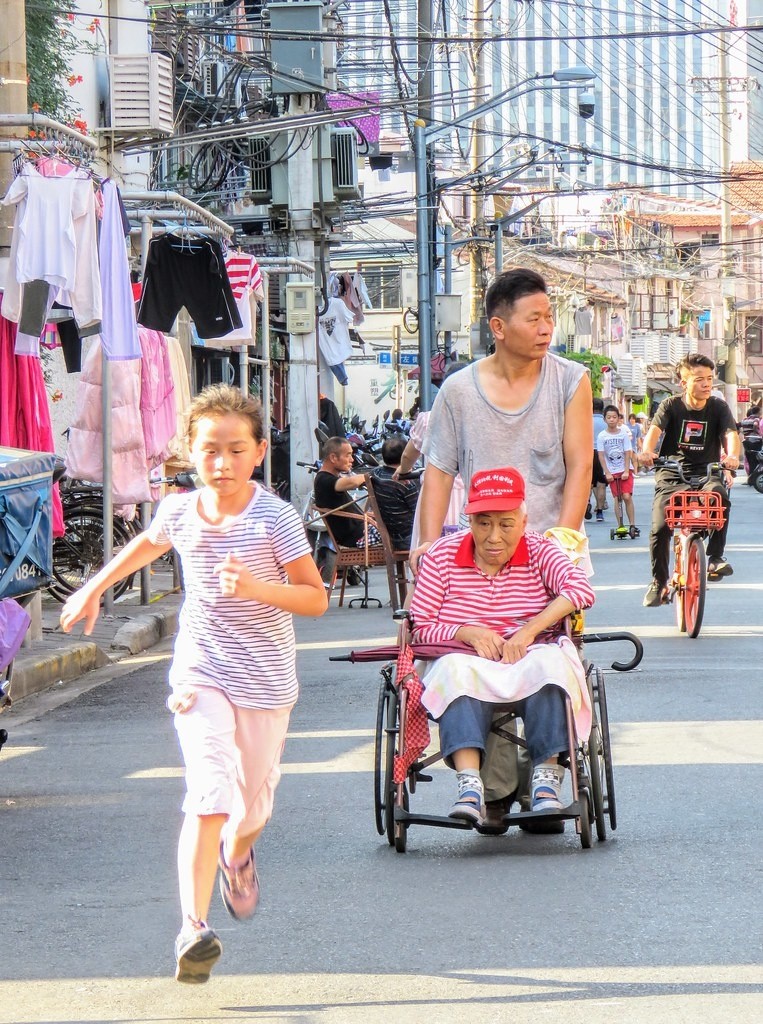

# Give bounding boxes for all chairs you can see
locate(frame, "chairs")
[313,504,410,609]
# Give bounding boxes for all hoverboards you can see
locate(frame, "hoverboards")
[610,476,641,541]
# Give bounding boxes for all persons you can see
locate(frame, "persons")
[584,398,662,533]
[408,461,595,827]
[362,404,428,553]
[741,407,763,484]
[635,353,742,606]
[47,371,331,988]
[312,434,388,549]
[394,361,480,647]
[400,267,595,838]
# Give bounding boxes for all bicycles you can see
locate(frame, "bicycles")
[646,455,744,639]
[45,475,141,608]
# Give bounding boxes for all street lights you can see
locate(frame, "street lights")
[494,190,592,281]
[414,65,598,411]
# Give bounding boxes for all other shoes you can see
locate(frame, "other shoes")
[448,788,487,829]
[584,504,592,519]
[638,471,646,475]
[519,808,566,833]
[631,473,640,478]
[530,785,564,812]
[476,786,519,834]
[616,533,628,540]
[596,513,605,521]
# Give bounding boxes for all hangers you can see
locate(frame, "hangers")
[150,212,258,263]
[11,125,113,186]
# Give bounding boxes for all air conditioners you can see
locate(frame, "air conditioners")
[203,61,226,98]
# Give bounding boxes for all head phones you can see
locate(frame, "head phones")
[578,93,595,119]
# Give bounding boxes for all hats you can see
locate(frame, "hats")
[637,412,649,419]
[464,467,525,515]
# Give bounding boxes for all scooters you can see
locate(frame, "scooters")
[741,435,763,493]
[251,404,410,590]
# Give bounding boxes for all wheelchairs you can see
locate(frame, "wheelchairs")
[372,555,616,853]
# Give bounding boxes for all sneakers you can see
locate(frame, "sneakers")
[707,556,733,582]
[174,915,223,984]
[217,837,261,920]
[642,578,670,607]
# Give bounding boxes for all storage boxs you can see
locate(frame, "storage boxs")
[0,446,56,600]
